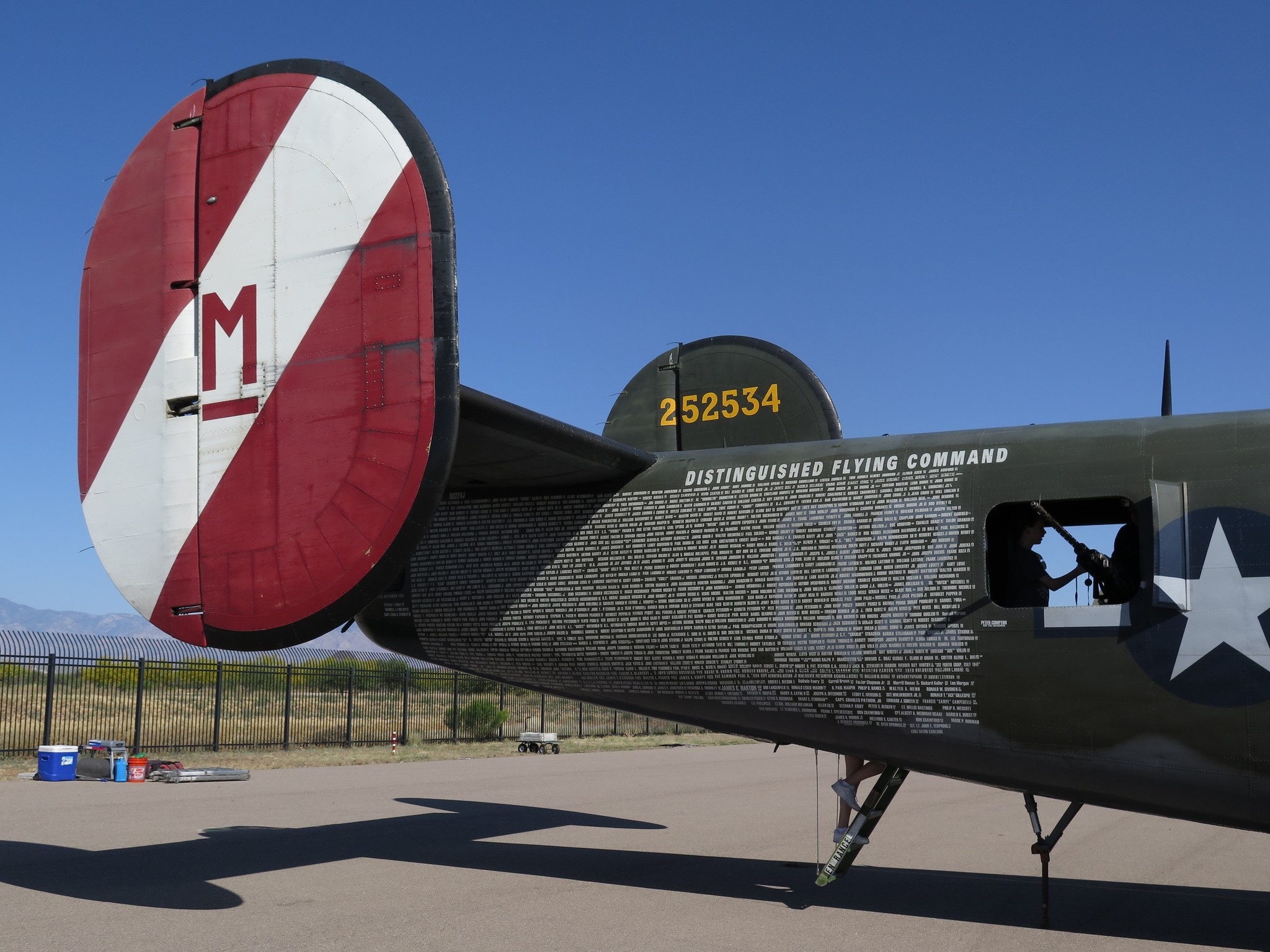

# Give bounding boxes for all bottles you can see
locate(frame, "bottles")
[115,757,126,782]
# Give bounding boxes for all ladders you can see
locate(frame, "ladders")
[149,767,250,784]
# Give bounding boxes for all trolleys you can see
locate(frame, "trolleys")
[515,732,564,754]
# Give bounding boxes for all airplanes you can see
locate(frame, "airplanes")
[78,57,1270,937]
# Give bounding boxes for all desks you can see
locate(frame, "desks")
[76,745,128,782]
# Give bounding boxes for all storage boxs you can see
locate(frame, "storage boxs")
[34,745,78,781]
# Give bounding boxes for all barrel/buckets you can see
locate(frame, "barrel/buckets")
[128,757,148,782]
